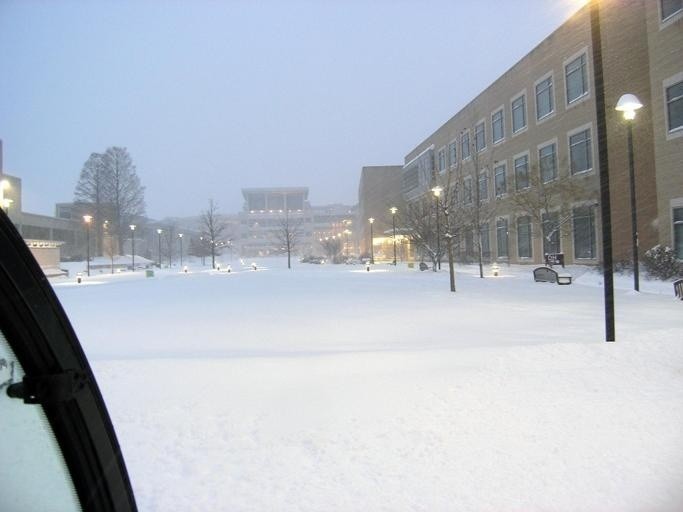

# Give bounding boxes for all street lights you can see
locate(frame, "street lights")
[319,229,352,265]
[128,222,137,272]
[177,232,234,271]
[366,217,375,263]
[82,215,93,278]
[0,198,15,217]
[388,205,396,263]
[614,93,646,291]
[155,229,164,268]
[431,185,441,273]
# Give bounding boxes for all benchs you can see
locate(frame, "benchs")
[673,280,682,299]
[533,266,571,285]
